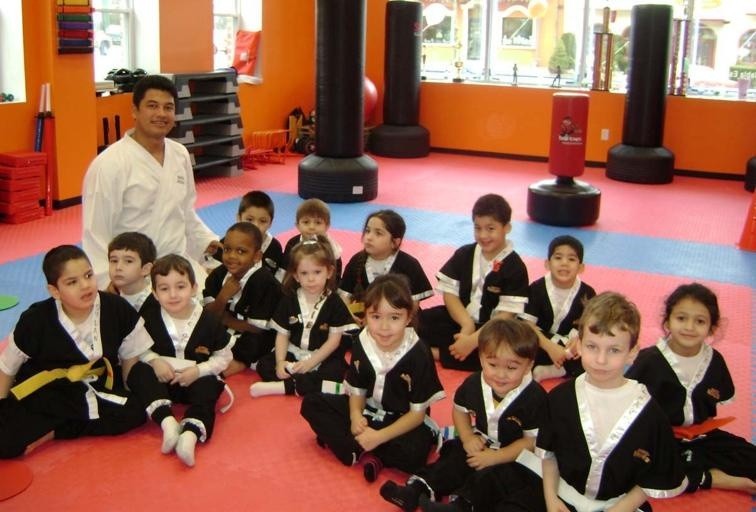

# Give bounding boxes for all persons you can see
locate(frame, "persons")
[623,283,756,495]
[552,65,562,86]
[513,64,518,83]
[126,253,234,467]
[104,231,159,313]
[0,245,154,458]
[382,291,689,512]
[81,76,224,304]
[516,235,597,382]
[200,190,448,484]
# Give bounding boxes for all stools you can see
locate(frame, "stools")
[241,130,294,170]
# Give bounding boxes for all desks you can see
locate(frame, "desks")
[160,69,245,181]
